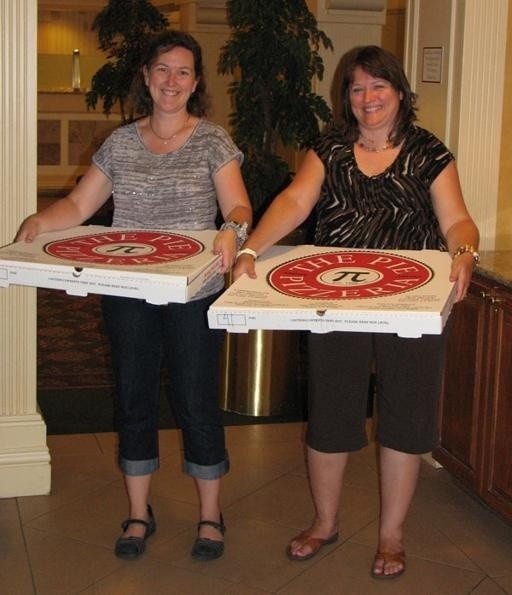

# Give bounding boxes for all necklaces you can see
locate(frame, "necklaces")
[355,139,395,151]
[150,112,189,144]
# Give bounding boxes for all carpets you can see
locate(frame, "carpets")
[35,283,169,392]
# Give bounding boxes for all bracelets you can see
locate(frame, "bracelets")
[451,244,478,267]
[219,220,249,248]
[234,249,259,260]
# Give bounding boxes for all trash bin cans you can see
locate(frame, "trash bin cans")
[217,330,303,420]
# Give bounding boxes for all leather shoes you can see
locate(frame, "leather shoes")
[192,511,226,561]
[113,504,156,559]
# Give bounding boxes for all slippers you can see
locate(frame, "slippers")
[286,526,338,560]
[370,548,407,577]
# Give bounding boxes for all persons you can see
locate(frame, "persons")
[14,30,252,563]
[229,43,479,582]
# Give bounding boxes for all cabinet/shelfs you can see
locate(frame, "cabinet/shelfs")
[431,273,512,527]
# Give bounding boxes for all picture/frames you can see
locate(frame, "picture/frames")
[421,46,443,83]
